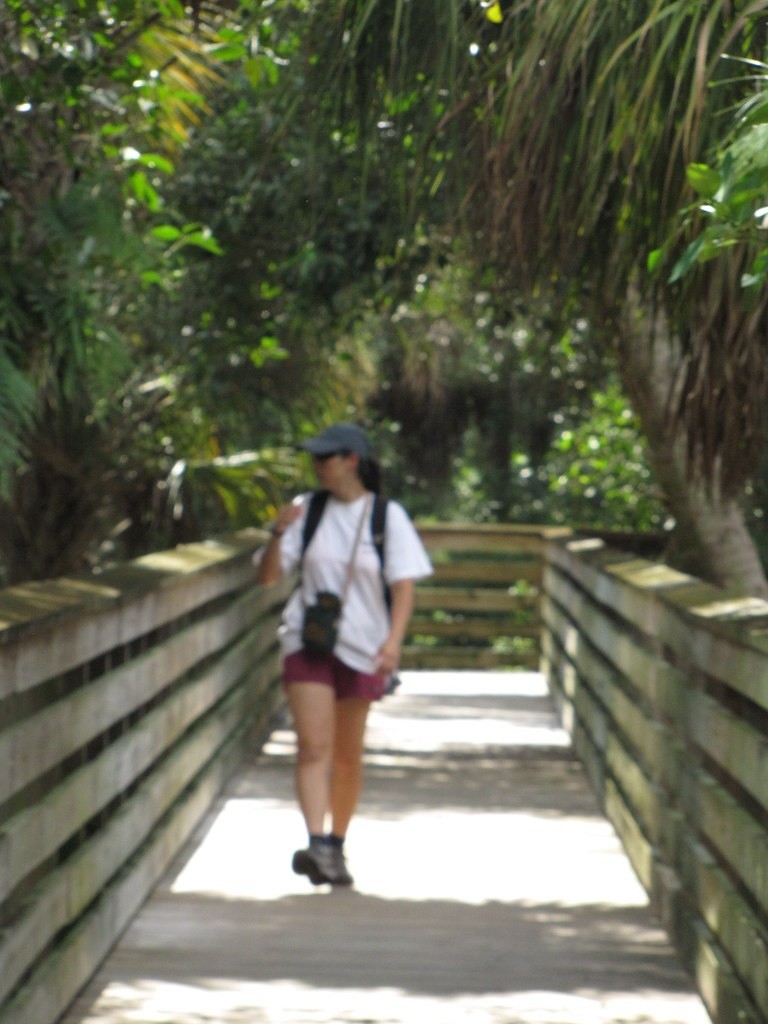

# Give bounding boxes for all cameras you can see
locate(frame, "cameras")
[299,590,339,662]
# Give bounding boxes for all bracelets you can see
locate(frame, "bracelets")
[268,523,283,537]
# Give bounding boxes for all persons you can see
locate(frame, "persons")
[260,424,433,889]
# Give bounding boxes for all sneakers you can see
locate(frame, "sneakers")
[292,844,352,885]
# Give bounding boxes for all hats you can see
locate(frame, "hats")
[300,424,371,457]
